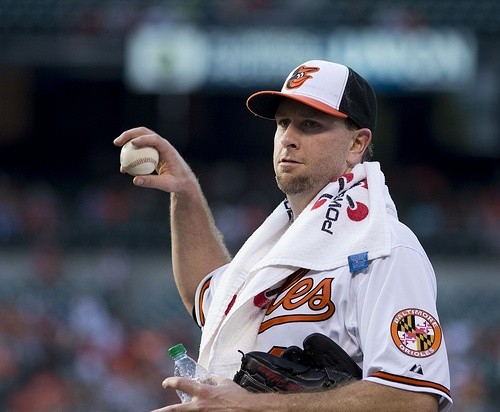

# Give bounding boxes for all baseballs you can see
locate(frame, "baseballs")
[120,138,159,176]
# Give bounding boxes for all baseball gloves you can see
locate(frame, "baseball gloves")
[233,333,363,394]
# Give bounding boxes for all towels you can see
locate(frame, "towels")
[195,161,399,382]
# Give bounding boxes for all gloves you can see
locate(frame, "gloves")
[233,333,363,393]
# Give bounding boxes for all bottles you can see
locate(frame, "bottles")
[169,344,214,404]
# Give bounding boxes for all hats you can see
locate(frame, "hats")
[246,60,376,131]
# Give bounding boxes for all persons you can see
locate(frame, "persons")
[113,60,453,412]
